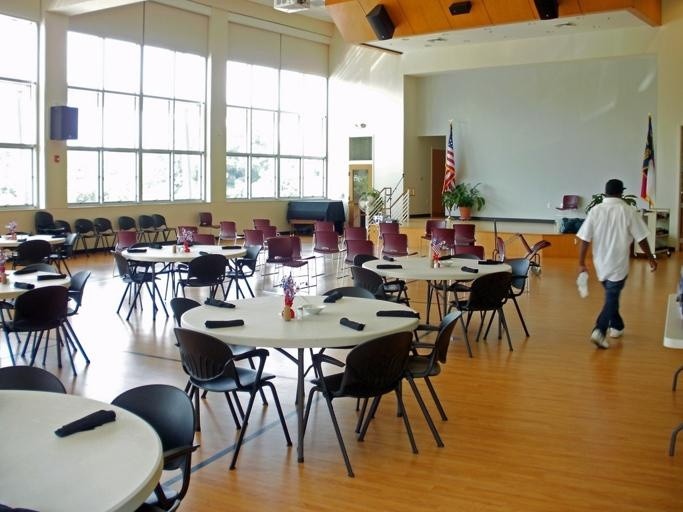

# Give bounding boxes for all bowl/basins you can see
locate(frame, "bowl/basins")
[302,303,325,314]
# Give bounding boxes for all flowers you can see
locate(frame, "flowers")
[280,273,298,302]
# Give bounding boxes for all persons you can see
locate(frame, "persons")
[574,178,657,349]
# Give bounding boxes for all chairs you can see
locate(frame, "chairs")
[169,296,270,431]
[555,194,578,211]
[1,286,77,380]
[446,270,515,359]
[359,310,465,447]
[6,208,553,290]
[111,383,198,512]
[173,328,290,471]
[294,286,376,408]
[33,271,93,365]
[172,253,228,318]
[424,254,479,324]
[349,265,419,343]
[355,254,410,306]
[11,264,58,351]
[298,329,419,479]
[108,250,168,321]
[478,259,532,343]
[2,366,68,394]
[223,244,262,302]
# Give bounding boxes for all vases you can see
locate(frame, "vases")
[281,305,293,322]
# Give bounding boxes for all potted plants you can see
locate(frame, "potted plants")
[442,183,486,221]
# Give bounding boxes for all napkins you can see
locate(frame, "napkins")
[54,407,116,437]
[202,318,246,328]
[376,310,418,319]
[206,296,236,309]
[339,316,366,331]
[322,292,344,304]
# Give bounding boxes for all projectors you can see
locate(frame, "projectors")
[273,0,310,13]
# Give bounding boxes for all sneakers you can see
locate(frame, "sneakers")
[591,329,608,349]
[611,326,625,338]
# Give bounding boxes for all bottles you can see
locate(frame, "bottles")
[296,307,302,320]
[0,266,6,283]
[282,304,291,322]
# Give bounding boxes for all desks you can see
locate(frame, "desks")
[662,292,682,456]
[361,256,514,333]
[123,244,247,316]
[182,295,417,462]
[2,271,72,362]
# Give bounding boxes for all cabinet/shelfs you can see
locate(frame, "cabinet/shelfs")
[635,208,672,259]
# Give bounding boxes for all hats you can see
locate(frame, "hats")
[606,179,626,195]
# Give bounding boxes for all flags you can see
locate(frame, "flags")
[641,113,654,209]
[440,119,455,194]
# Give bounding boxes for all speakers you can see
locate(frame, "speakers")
[534,0,558,20]
[50,106,78,139]
[365,4,395,40]
